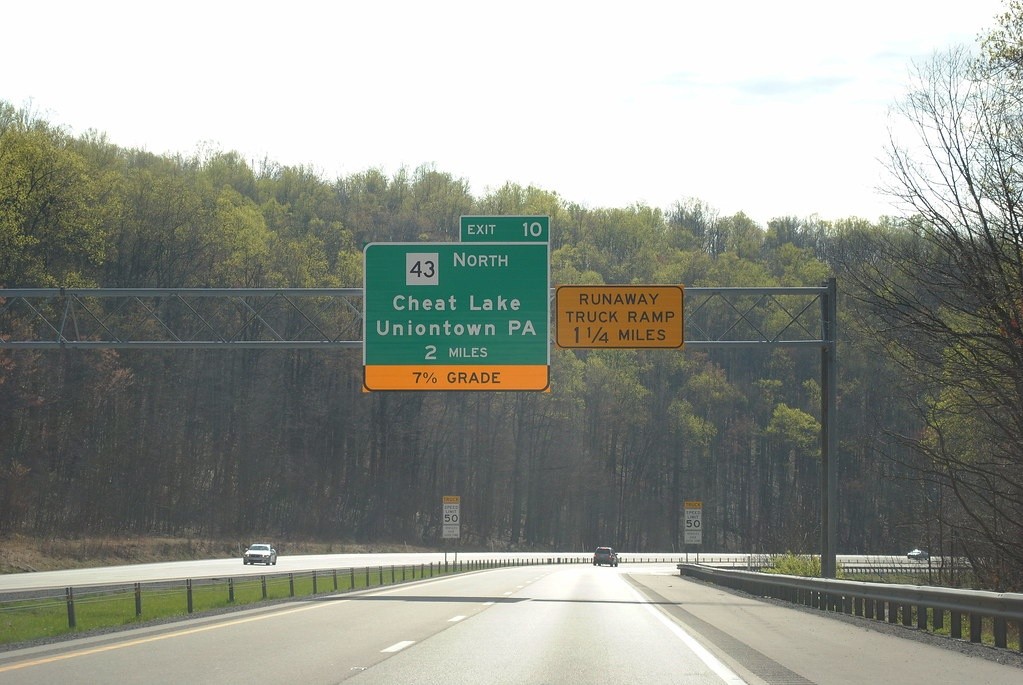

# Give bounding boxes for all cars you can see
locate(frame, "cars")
[244,543,277,566]
[907,549,929,560]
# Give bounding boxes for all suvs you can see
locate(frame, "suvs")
[593,547,618,567]
